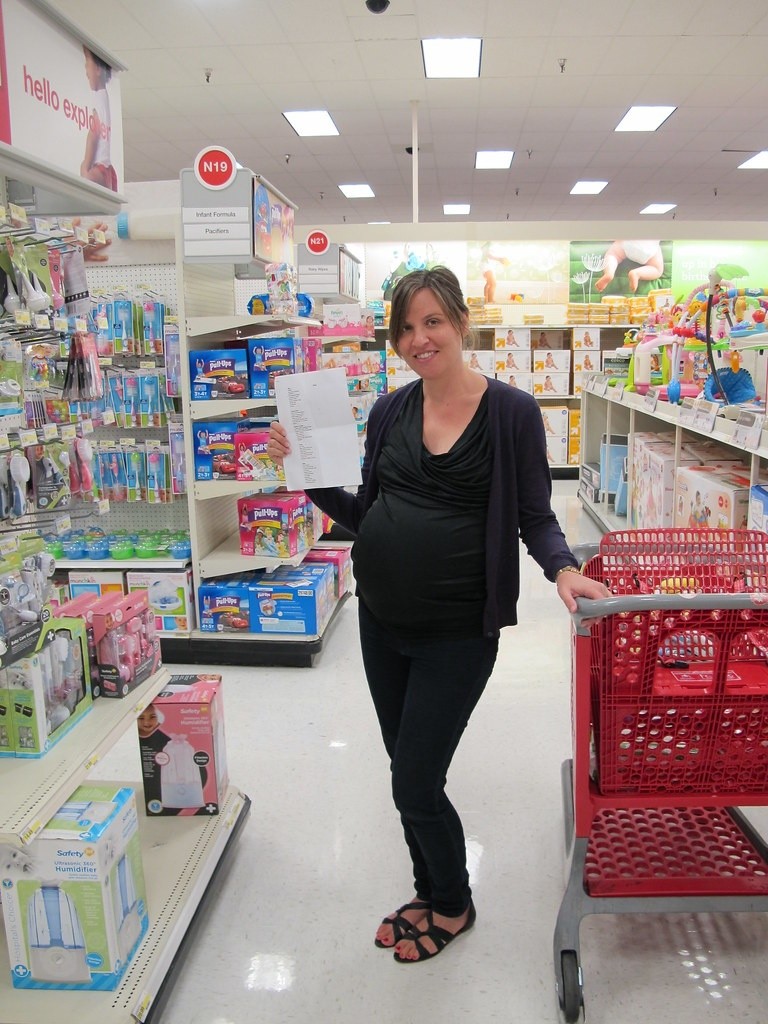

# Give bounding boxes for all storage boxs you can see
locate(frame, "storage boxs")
[190,329,768,636]
[0,588,230,993]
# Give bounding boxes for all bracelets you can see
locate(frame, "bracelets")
[557,566,580,577]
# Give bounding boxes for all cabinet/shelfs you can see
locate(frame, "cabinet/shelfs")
[188,315,354,667]
[385,323,640,469]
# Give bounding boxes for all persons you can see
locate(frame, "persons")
[81,45,117,192]
[137,704,171,801]
[547,445,555,462]
[542,412,555,434]
[196,359,205,376]
[545,352,558,369]
[253,346,267,372]
[197,429,211,455]
[506,330,519,347]
[352,407,360,419]
[238,443,246,457]
[509,375,517,386]
[584,332,594,347]
[170,674,221,685]
[59,218,111,260]
[469,353,483,370]
[539,332,551,348]
[270,267,626,963]
[240,505,249,529]
[544,376,557,392]
[584,355,593,370]
[364,316,374,335]
[691,491,705,515]
[506,353,519,369]
[480,247,505,302]
[595,240,664,291]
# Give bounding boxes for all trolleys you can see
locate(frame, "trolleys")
[552,527,768,1024]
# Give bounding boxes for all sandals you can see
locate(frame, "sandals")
[375,896,432,948]
[394,898,476,963]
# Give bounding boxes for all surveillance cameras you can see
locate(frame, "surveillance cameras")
[405,147,419,154]
[365,0,390,14]
